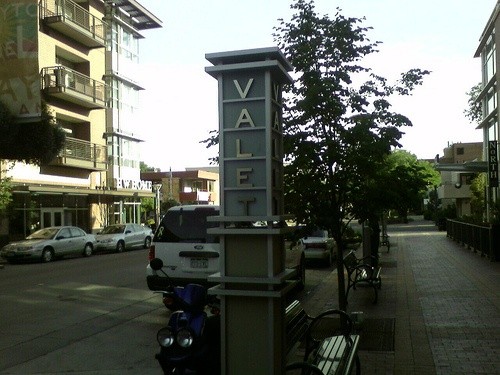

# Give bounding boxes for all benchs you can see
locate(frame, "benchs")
[281,298,363,375]
[343,250,383,305]
[378,232,391,252]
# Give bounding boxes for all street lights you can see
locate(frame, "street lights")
[153,184,161,225]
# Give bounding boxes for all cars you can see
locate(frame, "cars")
[3,226,97,262]
[146,205,306,290]
[302,229,338,268]
[97,223,154,252]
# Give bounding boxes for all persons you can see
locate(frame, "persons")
[208,193,212,201]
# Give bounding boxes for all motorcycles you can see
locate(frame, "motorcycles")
[150,258,220,375]
